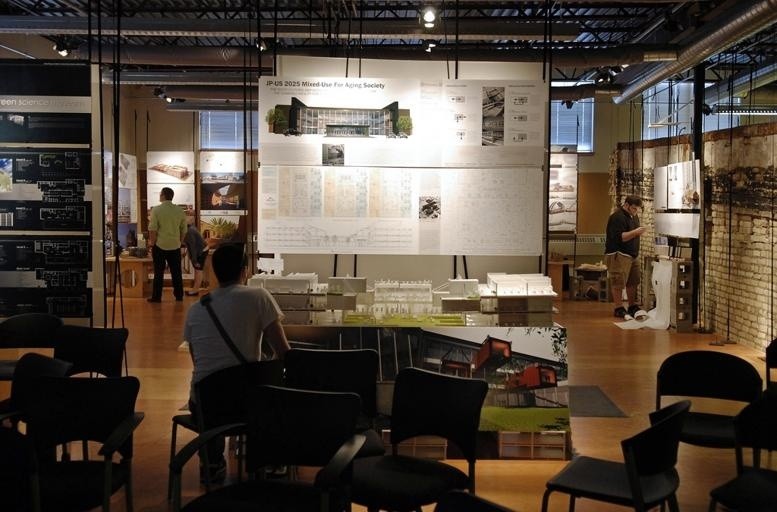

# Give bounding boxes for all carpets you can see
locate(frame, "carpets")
[559,380,629,425]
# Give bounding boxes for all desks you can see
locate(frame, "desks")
[105,252,154,298]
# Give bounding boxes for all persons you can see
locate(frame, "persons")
[183,222,208,297]
[145,186,185,302]
[180,243,290,415]
[604,197,648,320]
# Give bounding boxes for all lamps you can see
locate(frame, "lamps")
[420,39,438,54]
[52,41,79,57]
[153,87,166,100]
[710,104,777,116]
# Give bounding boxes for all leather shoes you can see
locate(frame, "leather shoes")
[185,291,199,296]
[147,298,161,303]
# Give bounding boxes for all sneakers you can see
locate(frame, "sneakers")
[628,305,643,320]
[614,307,628,318]
